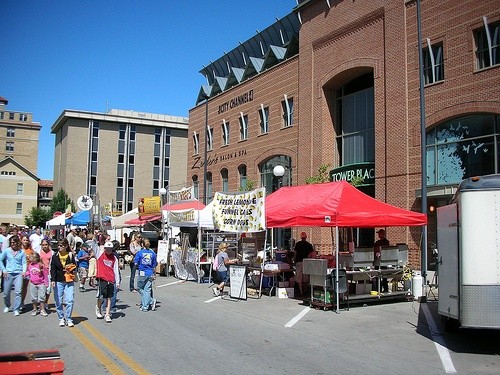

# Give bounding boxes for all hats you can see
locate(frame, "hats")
[301,232,307,239]
[376,229,385,233]
[103,241,114,248]
[71,226,77,230]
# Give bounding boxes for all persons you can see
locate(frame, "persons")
[49,238,78,327]
[372,228,390,293]
[213,242,236,296]
[123,233,157,311]
[292,232,316,296]
[0,222,122,322]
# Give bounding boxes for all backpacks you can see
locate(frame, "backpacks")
[213,254,223,271]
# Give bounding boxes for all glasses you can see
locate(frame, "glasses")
[58,244,66,248]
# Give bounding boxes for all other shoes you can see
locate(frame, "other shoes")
[45,309,50,314]
[20,306,26,311]
[151,299,157,311]
[142,308,147,312]
[136,300,142,306]
[96,312,103,318]
[79,287,87,292]
[212,287,219,297]
[14,310,19,316]
[104,315,111,322]
[40,310,48,317]
[32,309,37,316]
[131,289,138,293]
[4,306,9,312]
[37,307,40,313]
[139,305,143,311]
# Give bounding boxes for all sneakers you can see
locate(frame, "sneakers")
[59,319,65,326]
[65,320,75,327]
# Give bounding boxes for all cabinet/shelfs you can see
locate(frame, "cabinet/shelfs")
[201,233,238,260]
[310,268,413,309]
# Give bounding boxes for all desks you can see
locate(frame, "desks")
[264,268,294,299]
[184,261,213,286]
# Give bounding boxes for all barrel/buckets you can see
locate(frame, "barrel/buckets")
[275,250,288,263]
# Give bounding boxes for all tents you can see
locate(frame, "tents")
[110,207,140,241]
[169,200,241,285]
[45,211,75,229]
[124,201,205,225]
[265,181,429,313]
[65,210,85,228]
[71,211,112,227]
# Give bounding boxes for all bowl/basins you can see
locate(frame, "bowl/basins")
[370,291,377,295]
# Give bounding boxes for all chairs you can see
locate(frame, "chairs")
[427,271,438,301]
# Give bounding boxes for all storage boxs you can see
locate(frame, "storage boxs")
[265,263,290,270]
[351,283,372,294]
[262,275,273,289]
[276,288,294,299]
